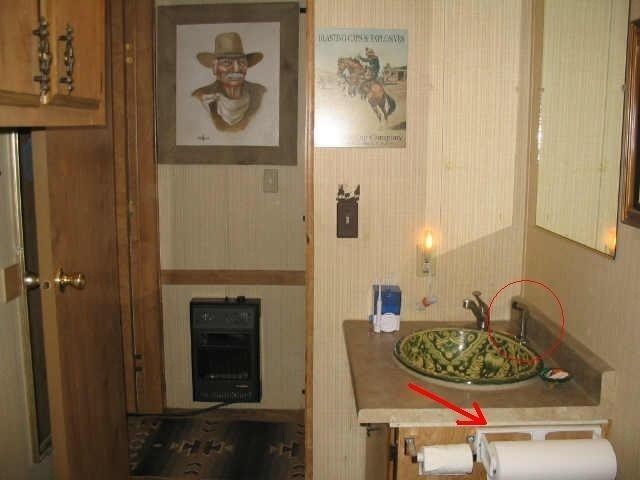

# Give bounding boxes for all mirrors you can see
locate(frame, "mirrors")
[535,0,631,259]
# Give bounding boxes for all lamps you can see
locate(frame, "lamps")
[417,232,436,278]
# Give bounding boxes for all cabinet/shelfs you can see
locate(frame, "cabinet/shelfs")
[0,0,105,127]
[360,422,612,480]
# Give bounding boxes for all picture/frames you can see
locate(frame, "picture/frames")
[157,1,299,164]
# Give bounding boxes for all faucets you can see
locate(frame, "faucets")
[461,290,490,332]
[395,326,544,389]
